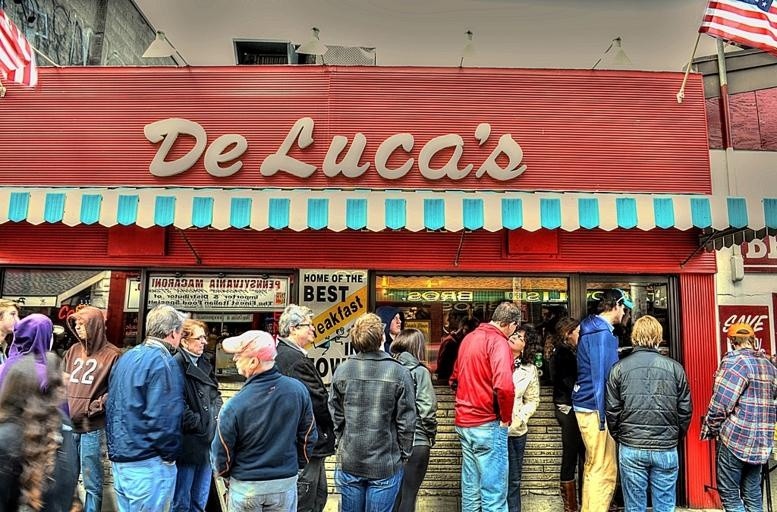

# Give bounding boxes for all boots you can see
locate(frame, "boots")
[560,478,580,512]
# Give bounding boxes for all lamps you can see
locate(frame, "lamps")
[296,26,327,64]
[139,29,191,69]
[455,27,479,71]
[591,36,632,72]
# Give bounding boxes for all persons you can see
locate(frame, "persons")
[329,308,435,511]
[208,305,334,511]
[546,317,583,512]
[571,286,628,511]
[503,324,540,511]
[446,301,523,511]
[605,316,693,510]
[435,315,481,384]
[2,295,224,512]
[698,322,776,512]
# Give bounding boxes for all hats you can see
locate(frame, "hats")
[222,329,277,361]
[728,322,755,337]
[598,287,633,309]
